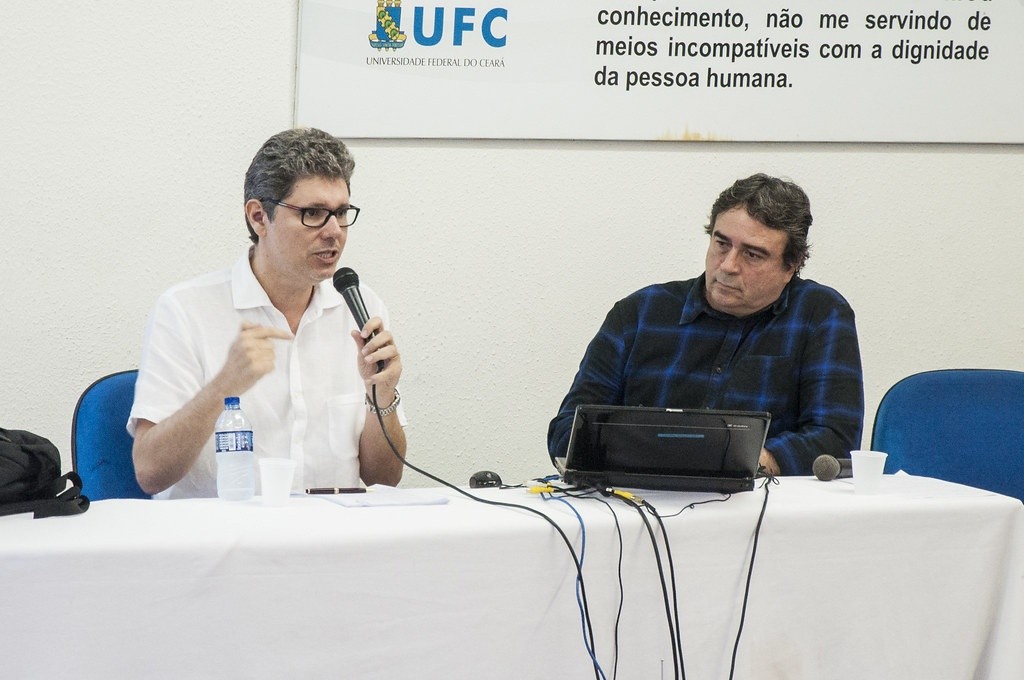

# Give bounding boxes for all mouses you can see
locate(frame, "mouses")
[469,470,502,489]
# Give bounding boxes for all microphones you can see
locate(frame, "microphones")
[332,267,384,370]
[812,454,853,481]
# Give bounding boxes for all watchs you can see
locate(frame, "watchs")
[364,388,400,416]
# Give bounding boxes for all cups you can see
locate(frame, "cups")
[850,450,889,494]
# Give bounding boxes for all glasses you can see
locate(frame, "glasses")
[260,197,361,228]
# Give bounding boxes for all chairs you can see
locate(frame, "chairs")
[869,368,1024,504]
[72,369,159,502]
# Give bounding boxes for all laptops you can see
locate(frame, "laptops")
[554,403,772,494]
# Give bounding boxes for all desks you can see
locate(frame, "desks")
[1,471,1022,680]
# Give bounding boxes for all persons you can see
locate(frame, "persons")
[126,128,406,499]
[546,173,863,476]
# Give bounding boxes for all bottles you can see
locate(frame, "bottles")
[212,394,262,512]
